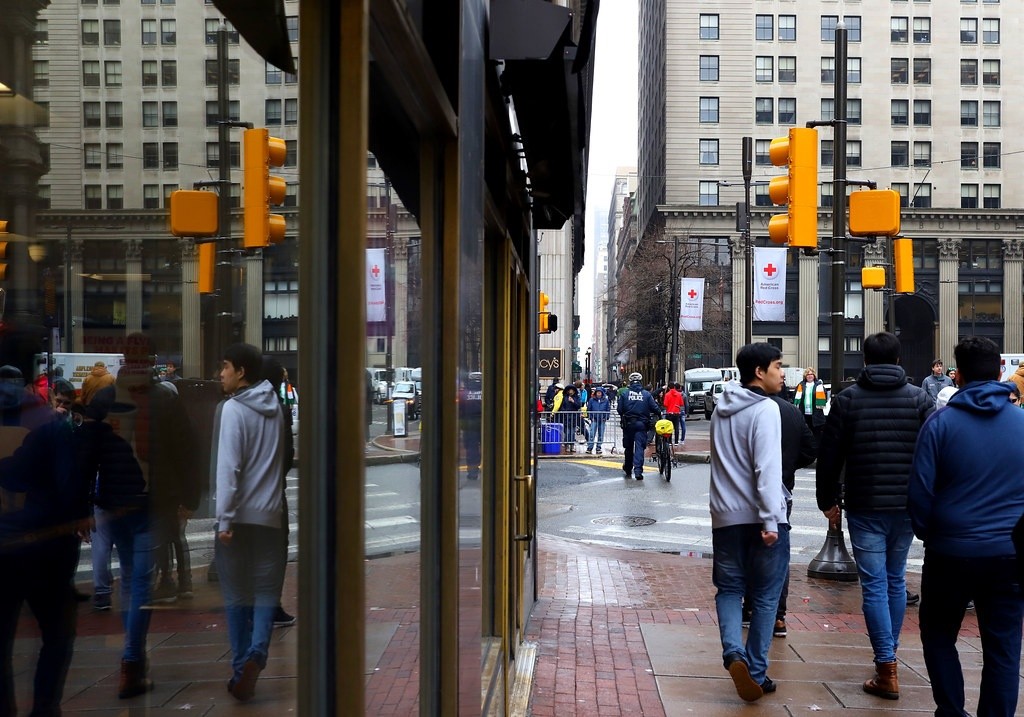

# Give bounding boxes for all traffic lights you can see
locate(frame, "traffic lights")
[540,292,550,332]
[243,128,287,249]
[768,127,817,250]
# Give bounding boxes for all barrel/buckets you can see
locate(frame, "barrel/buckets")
[540,423,564,455]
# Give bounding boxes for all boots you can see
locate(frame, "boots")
[119,660,153,698]
[863,657,899,700]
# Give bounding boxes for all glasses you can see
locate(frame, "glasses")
[55,397,72,405]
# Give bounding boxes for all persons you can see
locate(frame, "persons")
[213,343,287,702]
[794,367,827,463]
[1010,361,1024,399]
[1001,381,1024,409]
[617,372,662,480]
[945,367,957,389]
[816,331,935,701]
[617,381,629,424]
[663,382,688,446]
[710,342,792,703]
[922,359,955,405]
[0,332,205,717]
[645,384,667,444]
[460,372,482,480]
[906,336,1024,717]
[536,377,616,454]
[742,390,817,635]
[250,360,295,624]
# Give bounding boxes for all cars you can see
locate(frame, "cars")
[366,367,422,420]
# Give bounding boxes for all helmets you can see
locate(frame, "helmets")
[628,372,642,382]
[554,383,564,390]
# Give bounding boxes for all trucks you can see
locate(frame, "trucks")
[683,367,739,415]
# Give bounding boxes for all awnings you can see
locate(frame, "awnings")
[572,363,582,373]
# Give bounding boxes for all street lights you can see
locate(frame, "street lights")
[587,347,592,383]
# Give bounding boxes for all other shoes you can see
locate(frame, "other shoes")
[674,444,678,446]
[91,593,112,610]
[723,653,763,702]
[679,441,684,445]
[635,474,643,480]
[596,450,602,454]
[906,590,919,603]
[742,607,751,624]
[227,653,264,701]
[565,444,570,452]
[153,581,175,598]
[571,445,576,452]
[622,464,632,475]
[175,581,194,597]
[761,676,776,693]
[966,603,974,609]
[271,603,296,626]
[773,620,787,636]
[669,444,672,446]
[586,450,592,453]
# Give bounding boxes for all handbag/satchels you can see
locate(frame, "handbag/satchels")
[580,403,588,417]
[655,419,673,435]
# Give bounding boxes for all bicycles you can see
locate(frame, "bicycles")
[649,412,674,482]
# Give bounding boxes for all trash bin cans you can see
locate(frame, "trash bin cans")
[542,423,563,454]
[392,397,409,437]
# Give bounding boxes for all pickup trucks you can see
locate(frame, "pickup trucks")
[704,382,725,420]
